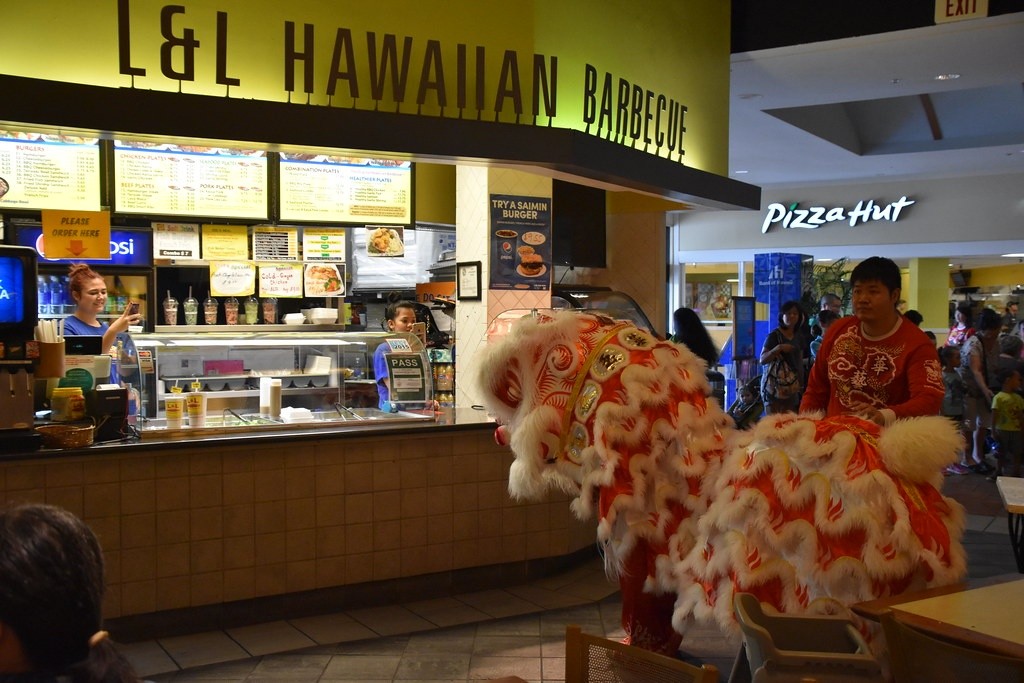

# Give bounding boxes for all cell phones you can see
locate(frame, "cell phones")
[128,303,139,321]
[412,322,426,348]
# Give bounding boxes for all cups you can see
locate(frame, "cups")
[35,343,66,378]
[243,298,259,324]
[163,297,179,325]
[270,379,281,420]
[495,230,518,275]
[164,397,184,428]
[186,393,207,426]
[224,298,239,324]
[183,297,199,325]
[202,298,218,325]
[359,313,366,326]
[262,298,277,324]
[260,378,270,418]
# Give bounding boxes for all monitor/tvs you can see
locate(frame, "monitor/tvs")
[0,244,39,342]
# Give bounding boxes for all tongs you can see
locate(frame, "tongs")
[222,409,251,426]
[333,401,364,421]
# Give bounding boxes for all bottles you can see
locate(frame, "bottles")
[51,387,85,423]
[434,392,454,407]
[343,299,352,324]
[38,276,74,314]
[433,364,454,390]
[354,358,361,380]
[126,383,137,426]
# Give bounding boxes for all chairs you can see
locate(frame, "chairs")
[564,591,1024,683]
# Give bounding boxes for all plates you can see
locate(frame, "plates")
[516,264,547,277]
[367,229,404,256]
[521,232,546,245]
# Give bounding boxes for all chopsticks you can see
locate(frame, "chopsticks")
[34,318,64,342]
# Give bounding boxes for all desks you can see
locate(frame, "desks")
[849,572,1024,660]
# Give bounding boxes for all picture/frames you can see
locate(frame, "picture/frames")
[455,261,481,301]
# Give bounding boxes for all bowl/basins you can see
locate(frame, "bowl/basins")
[711,301,731,318]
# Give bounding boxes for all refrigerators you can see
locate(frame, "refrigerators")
[17,219,157,419]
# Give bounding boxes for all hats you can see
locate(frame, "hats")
[1007,301,1020,307]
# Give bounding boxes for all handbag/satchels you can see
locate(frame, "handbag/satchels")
[764,331,800,399]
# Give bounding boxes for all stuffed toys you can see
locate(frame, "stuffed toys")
[477,305,967,656]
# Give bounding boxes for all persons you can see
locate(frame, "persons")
[733,385,765,430]
[375,300,440,413]
[903,300,1024,482]
[0,497,141,683]
[668,307,722,371]
[760,292,842,416]
[801,256,945,426]
[62,260,140,416]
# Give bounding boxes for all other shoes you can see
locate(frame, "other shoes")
[947,464,969,475]
[985,471,1002,481]
[977,459,996,470]
[961,462,984,473]
